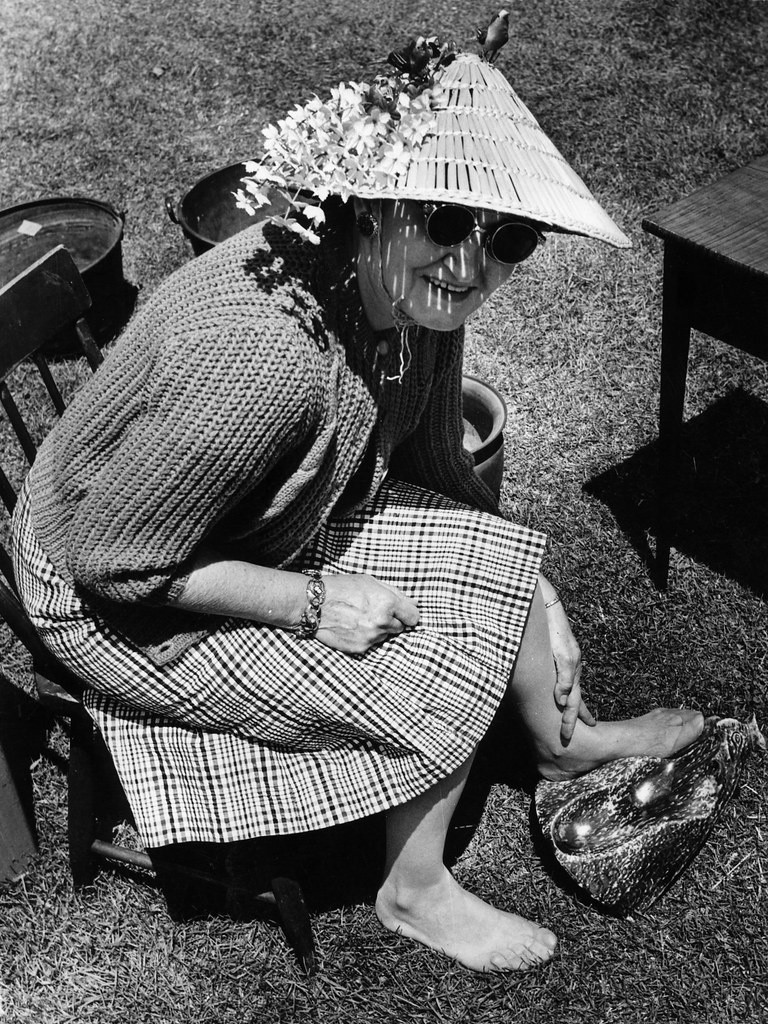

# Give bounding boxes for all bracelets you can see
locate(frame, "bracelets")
[544,595,560,609]
[293,570,326,642]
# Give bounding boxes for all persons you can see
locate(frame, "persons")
[13,82,706,976]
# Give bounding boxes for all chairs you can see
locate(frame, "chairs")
[3,243,329,980]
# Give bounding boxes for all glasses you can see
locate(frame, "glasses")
[418,200,546,266]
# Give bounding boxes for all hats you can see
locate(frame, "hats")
[278,48,636,251]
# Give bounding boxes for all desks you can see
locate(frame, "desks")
[640,146,767,592]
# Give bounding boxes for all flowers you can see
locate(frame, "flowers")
[228,40,467,230]
